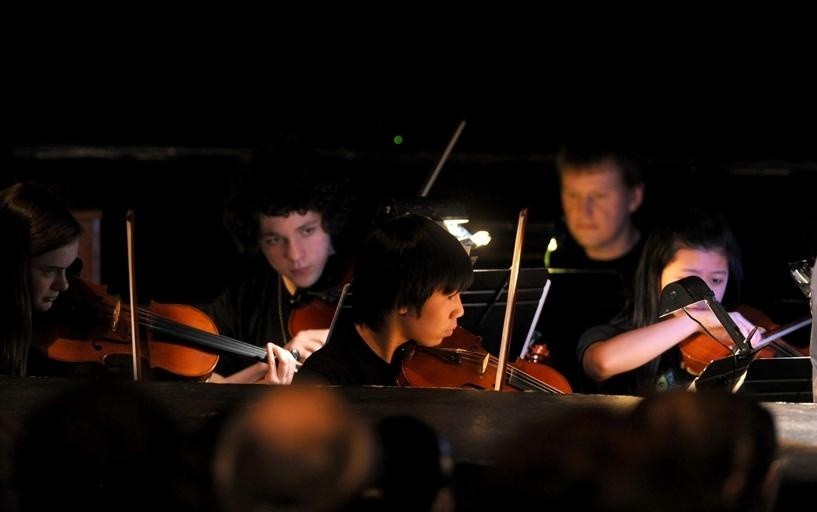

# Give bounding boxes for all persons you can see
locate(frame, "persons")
[289,209,477,390]
[1,181,300,386]
[540,211,767,394]
[203,182,351,380]
[517,122,654,341]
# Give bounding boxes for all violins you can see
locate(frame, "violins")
[396,324,573,394]
[679,306,810,376]
[47,276,303,381]
[288,265,354,338]
